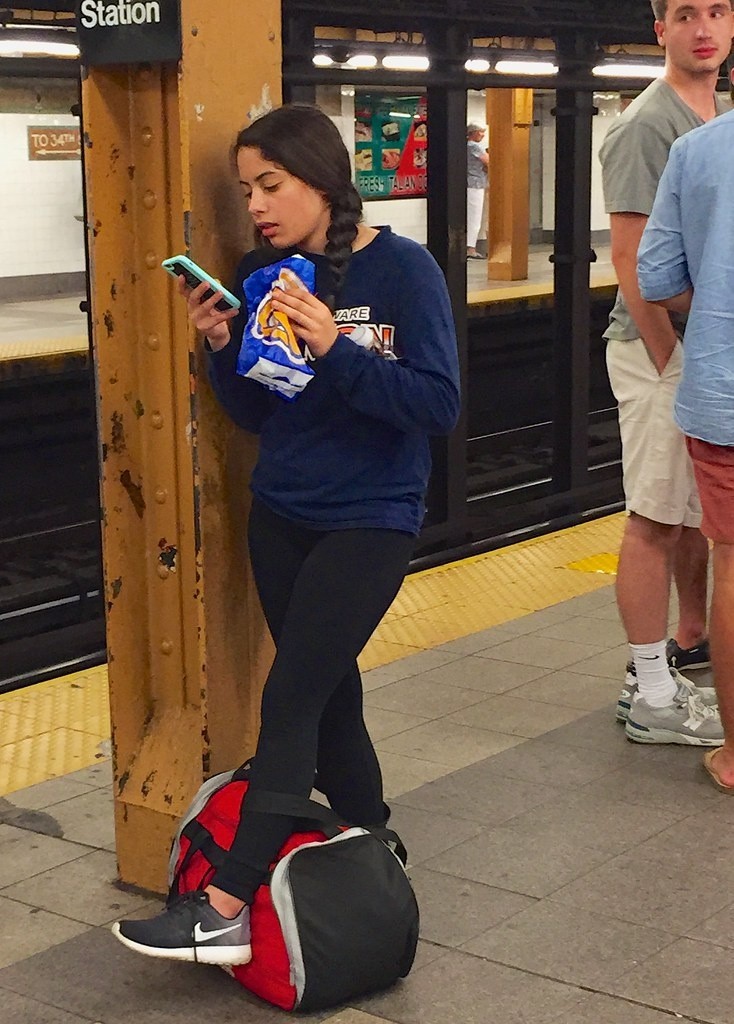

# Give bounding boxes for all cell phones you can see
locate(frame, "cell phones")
[162,255,241,312]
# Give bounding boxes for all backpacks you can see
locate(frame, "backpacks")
[166,757,420,1011]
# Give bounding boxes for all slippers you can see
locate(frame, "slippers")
[703,747,734,794]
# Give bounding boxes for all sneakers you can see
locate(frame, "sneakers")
[625,681,726,745]
[626,638,712,680]
[111,888,252,966]
[617,666,719,722]
[466,252,487,261]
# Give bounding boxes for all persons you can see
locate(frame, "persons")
[112,106,459,965]
[466,121,492,259]
[598,0,733,747]
[634,109,734,791]
[623,487,711,675]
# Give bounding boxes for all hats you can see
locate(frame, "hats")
[467,121,487,131]
